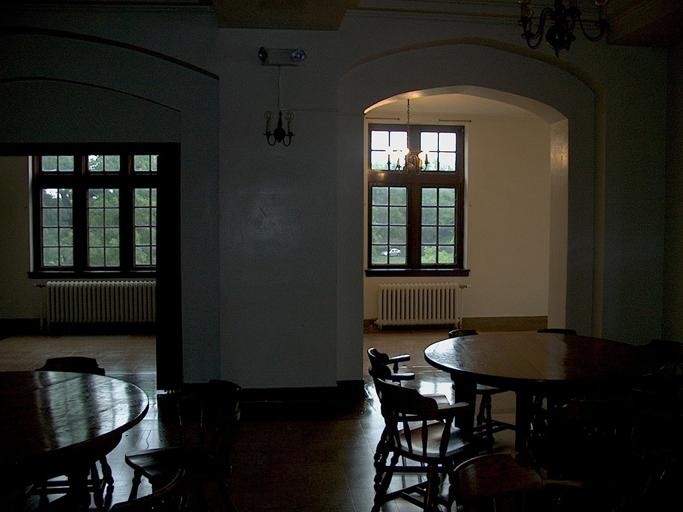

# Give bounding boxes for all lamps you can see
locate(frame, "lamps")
[521,0,607,57]
[385,98,428,176]
[263,65,294,147]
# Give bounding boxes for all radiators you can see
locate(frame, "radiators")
[376,282,462,328]
[45,281,156,331]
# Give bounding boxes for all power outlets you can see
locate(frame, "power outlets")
[463,278,471,288]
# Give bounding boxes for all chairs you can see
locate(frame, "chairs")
[448,329,501,420]
[430,447,584,510]
[110,379,241,510]
[34,356,105,376]
[366,364,472,510]
[367,346,452,466]
[511,329,682,486]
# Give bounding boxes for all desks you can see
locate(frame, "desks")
[418,332,618,465]
